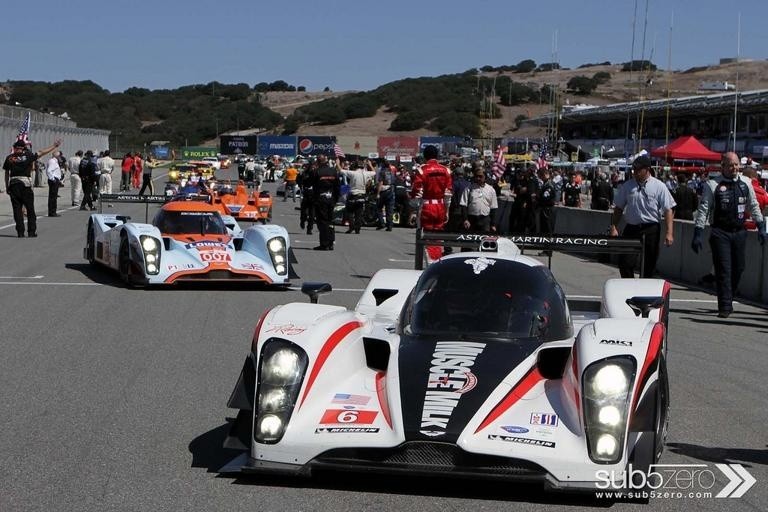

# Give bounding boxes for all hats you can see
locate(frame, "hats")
[628,156,650,168]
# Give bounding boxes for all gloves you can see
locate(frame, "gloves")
[691,226,703,254]
[756,223,766,245]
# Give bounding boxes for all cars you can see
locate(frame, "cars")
[167,153,310,182]
[276,181,312,197]
[311,188,421,224]
[697,81,735,90]
[222,228,671,504]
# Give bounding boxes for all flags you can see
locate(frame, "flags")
[16,112,31,143]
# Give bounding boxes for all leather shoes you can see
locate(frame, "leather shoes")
[718,311,728,317]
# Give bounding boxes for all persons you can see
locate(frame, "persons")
[237,144,768,319]
[3,136,216,239]
